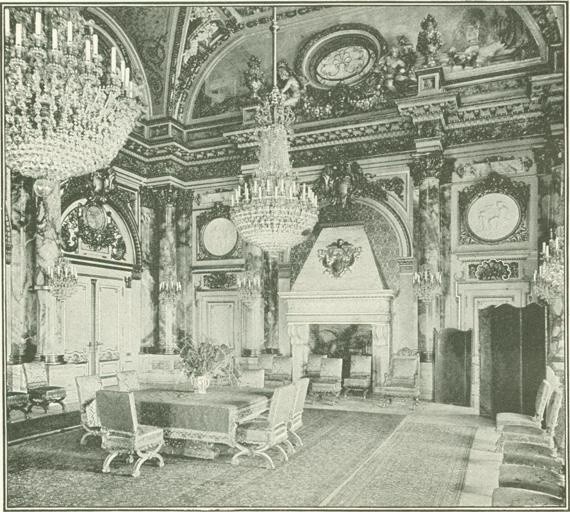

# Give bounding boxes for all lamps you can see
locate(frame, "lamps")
[535,168,567,318]
[228,7,320,268]
[0,3,147,199]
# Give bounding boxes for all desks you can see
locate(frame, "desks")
[135,383,283,460]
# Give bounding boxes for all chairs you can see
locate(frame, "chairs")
[309,358,343,402]
[96,390,165,478]
[261,378,309,448]
[301,355,328,380]
[496,380,565,509]
[117,371,141,391]
[5,366,26,424]
[344,356,373,399]
[24,361,65,414]
[266,355,293,389]
[232,383,294,469]
[77,375,103,445]
[381,356,422,408]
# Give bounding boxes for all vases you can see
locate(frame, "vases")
[190,374,213,394]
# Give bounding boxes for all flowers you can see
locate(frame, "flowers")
[174,338,240,384]
